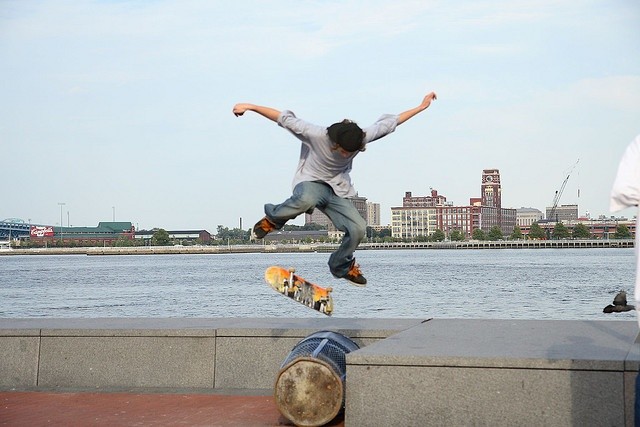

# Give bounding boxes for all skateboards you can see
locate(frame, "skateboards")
[265,266,333,317]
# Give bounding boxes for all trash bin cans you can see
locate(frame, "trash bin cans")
[275,330,361,427]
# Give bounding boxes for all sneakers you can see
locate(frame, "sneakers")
[253,217,273,240]
[343,257,366,284]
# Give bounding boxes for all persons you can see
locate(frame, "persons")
[233,92,437,287]
